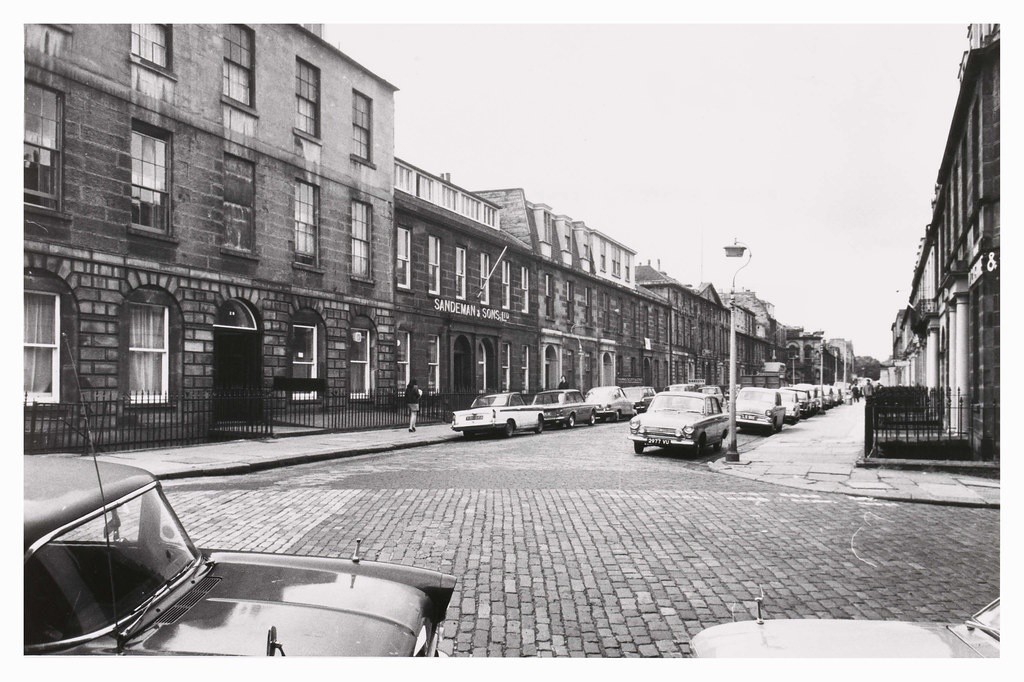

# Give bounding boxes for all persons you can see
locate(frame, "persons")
[559,375,569,389]
[852,379,883,403]
[405,379,420,432]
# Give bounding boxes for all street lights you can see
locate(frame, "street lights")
[725,238,752,462]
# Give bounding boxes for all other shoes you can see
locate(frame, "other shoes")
[409,426,416,432]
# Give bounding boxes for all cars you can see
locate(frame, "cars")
[450,392,544,439]
[689,593,1000,660]
[530,389,597,429]
[584,386,636,423]
[665,384,723,396]
[24,455,457,655]
[778,382,849,424]
[735,387,786,433]
[623,386,657,413]
[627,391,729,457]
